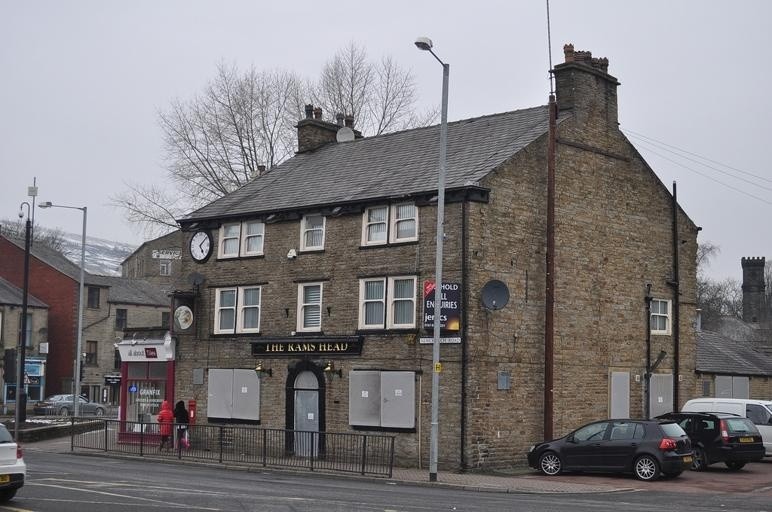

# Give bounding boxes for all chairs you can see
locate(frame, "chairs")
[612,427,642,440]
[686,421,691,427]
[703,422,708,427]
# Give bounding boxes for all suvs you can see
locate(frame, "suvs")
[652,411,765,473]
[0,423,27,503]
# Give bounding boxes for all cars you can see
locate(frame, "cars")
[526,418,692,482]
[33,394,107,418]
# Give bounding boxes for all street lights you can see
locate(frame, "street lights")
[17,202,30,421]
[38,201,88,417]
[414,35,451,485]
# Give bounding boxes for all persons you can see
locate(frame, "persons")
[174,400,191,450]
[157,401,174,451]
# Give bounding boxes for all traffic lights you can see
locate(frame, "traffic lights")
[2,348,17,382]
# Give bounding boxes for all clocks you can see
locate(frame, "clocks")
[190,230,214,263]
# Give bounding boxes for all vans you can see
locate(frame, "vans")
[680,396,771,460]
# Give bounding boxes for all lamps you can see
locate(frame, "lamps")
[254,360,272,378]
[324,361,342,385]
[113,331,146,351]
